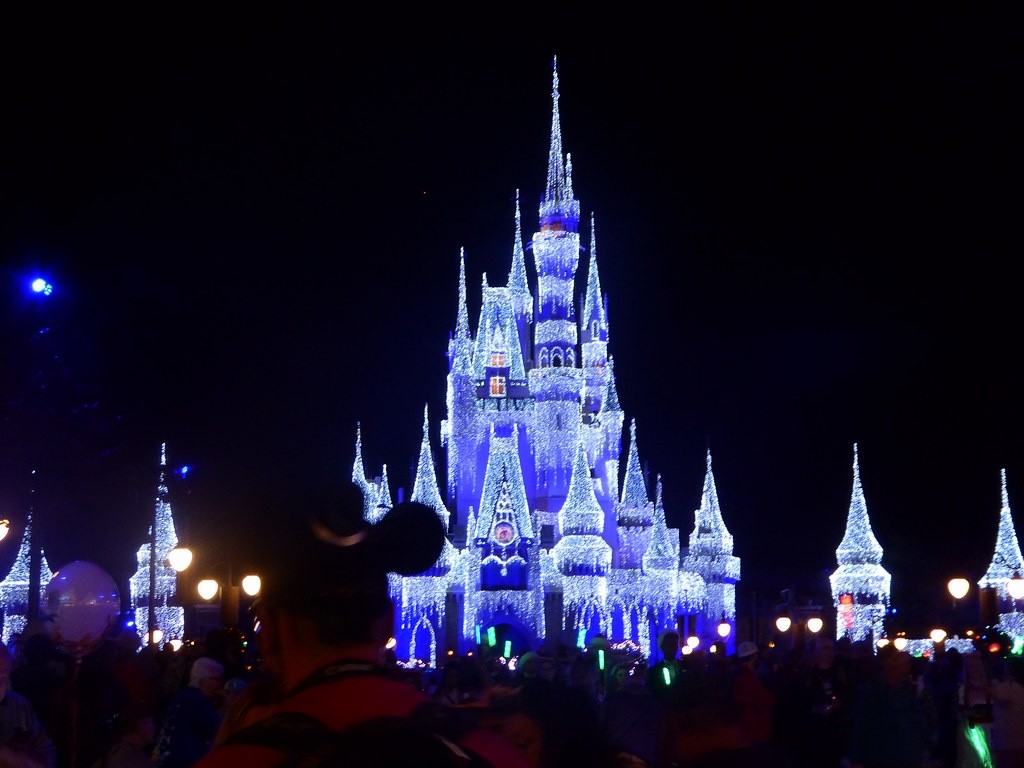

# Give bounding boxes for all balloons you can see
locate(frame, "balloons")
[42,560,121,660]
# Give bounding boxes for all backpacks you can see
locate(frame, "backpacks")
[217,699,497,767]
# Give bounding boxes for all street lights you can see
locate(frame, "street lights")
[182,466,188,611]
[22,273,55,625]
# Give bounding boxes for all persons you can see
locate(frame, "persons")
[0,497,1024,768]
